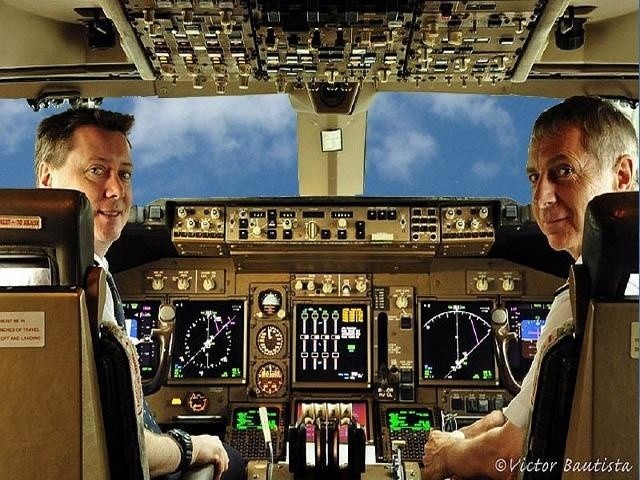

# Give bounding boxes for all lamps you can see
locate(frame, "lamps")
[74,5,117,52]
[555,5,597,50]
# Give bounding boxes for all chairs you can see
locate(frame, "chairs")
[526,191,639,477]
[1,189,151,479]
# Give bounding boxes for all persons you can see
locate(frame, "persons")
[420,96,638,479]
[32,108,246,479]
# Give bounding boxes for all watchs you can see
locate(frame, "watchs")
[167,427,194,475]
